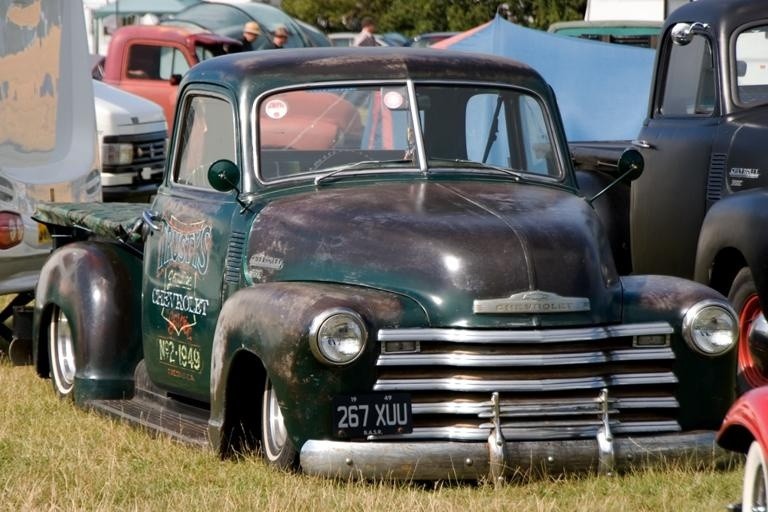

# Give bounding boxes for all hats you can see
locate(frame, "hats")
[268,26,289,38]
[242,21,260,36]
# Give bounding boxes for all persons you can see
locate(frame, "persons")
[227,21,262,53]
[353,16,376,46]
[263,25,295,50]
